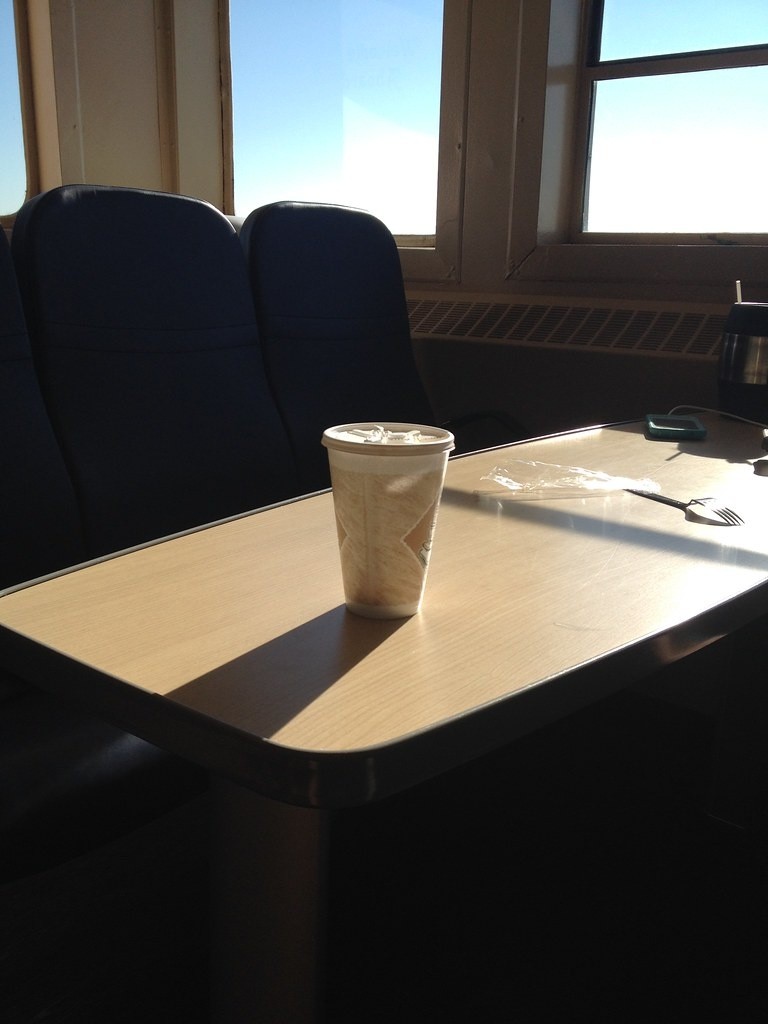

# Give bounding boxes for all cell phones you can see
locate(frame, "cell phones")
[645,414,707,439]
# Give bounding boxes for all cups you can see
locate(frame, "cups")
[321,421,455,619]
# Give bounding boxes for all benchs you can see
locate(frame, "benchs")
[1,183,438,890]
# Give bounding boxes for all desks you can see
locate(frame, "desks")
[0,412,767,1024]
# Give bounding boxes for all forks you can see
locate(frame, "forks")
[619,489,745,527]
